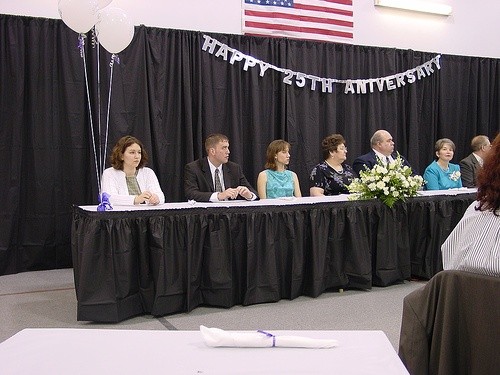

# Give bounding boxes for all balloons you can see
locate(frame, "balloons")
[58,0,98,34]
[95,7,134,54]
[97,0,112,9]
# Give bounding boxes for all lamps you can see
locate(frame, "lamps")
[374,0,453,16]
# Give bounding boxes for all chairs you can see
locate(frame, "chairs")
[397,270,499,375]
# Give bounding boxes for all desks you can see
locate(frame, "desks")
[71,187,480,322]
[0,328,410,375]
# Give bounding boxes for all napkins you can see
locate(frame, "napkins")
[200,325,339,348]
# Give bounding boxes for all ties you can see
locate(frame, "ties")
[386,157,390,163]
[215,169,223,192]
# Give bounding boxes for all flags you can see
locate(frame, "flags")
[241,0,353,40]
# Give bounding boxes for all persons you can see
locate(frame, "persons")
[353,130,423,191]
[441,134,500,278]
[183,132,260,202]
[458,135,492,188]
[309,134,358,197]
[100,136,165,206]
[257,140,301,199]
[423,138,463,191]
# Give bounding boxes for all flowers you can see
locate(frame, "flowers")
[448,170,461,181]
[344,150,429,208]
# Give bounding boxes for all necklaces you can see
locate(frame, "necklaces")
[325,160,343,174]
